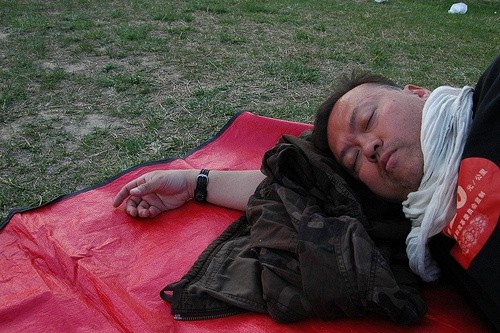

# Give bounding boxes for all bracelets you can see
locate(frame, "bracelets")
[193,168,210,202]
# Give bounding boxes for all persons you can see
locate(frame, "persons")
[111,53,500,333]
